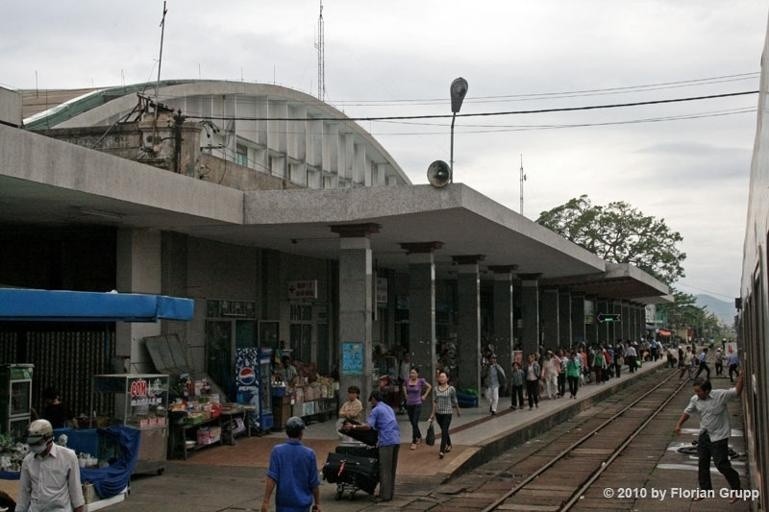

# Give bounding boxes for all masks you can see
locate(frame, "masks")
[29,438,53,454]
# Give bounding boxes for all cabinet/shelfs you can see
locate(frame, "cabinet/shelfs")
[272,395,294,432]
[301,390,339,426]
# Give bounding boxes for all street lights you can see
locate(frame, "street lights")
[445,76,469,183]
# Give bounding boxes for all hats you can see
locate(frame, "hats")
[368,390,377,402]
[27,419,52,443]
[286,416,307,429]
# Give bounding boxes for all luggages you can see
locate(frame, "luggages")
[336,440,379,459]
[337,415,378,446]
[322,452,381,494]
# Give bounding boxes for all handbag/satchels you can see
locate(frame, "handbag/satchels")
[426,419,436,446]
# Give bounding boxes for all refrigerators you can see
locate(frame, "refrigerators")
[234,347,275,438]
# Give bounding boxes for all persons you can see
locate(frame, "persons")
[525,336,663,411]
[396,351,414,415]
[691,350,699,375]
[41,387,76,430]
[694,348,710,381]
[481,353,506,415]
[403,367,431,450]
[678,346,684,369]
[339,386,363,441]
[715,349,726,376]
[674,367,746,504]
[482,348,491,367]
[510,362,524,410]
[14,418,85,512]
[678,346,692,381]
[261,416,321,512]
[273,355,299,386]
[728,351,740,385]
[667,344,676,369]
[343,391,401,501]
[429,372,461,457]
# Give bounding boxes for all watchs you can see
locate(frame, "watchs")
[353,425,357,431]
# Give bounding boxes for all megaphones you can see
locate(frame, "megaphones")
[426,159,451,187]
